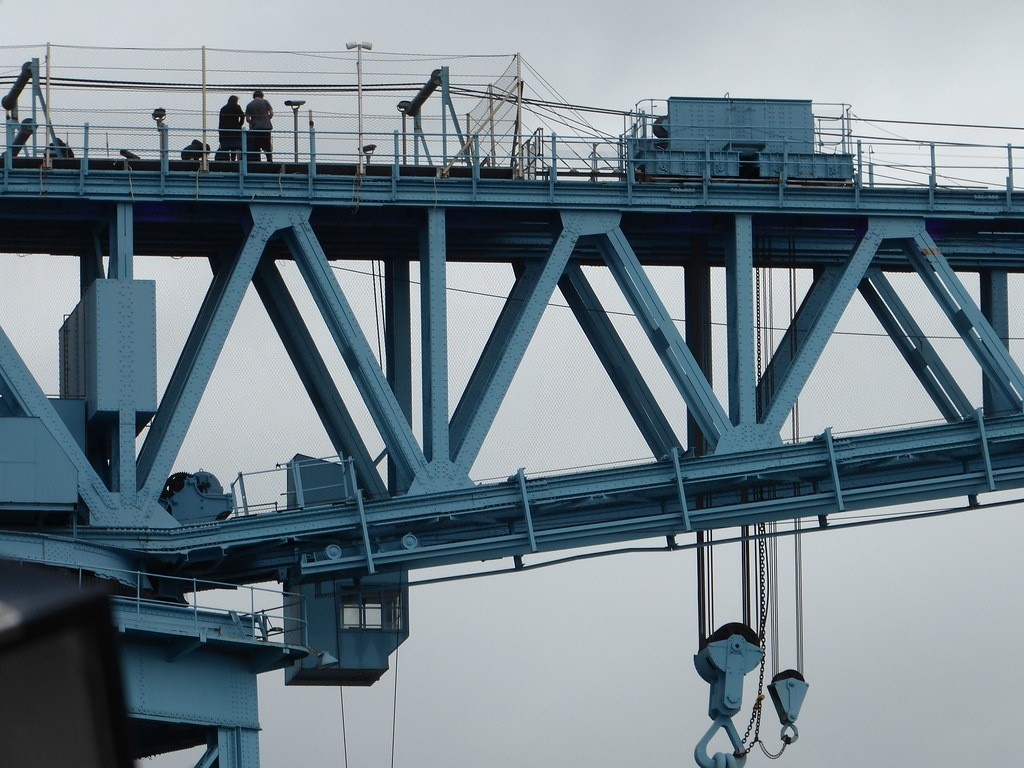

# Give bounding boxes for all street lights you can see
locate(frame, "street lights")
[347,41,374,176]
[285,98,305,163]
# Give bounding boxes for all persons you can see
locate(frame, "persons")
[246,91,273,163]
[219,96,245,161]
[181,140,210,161]
